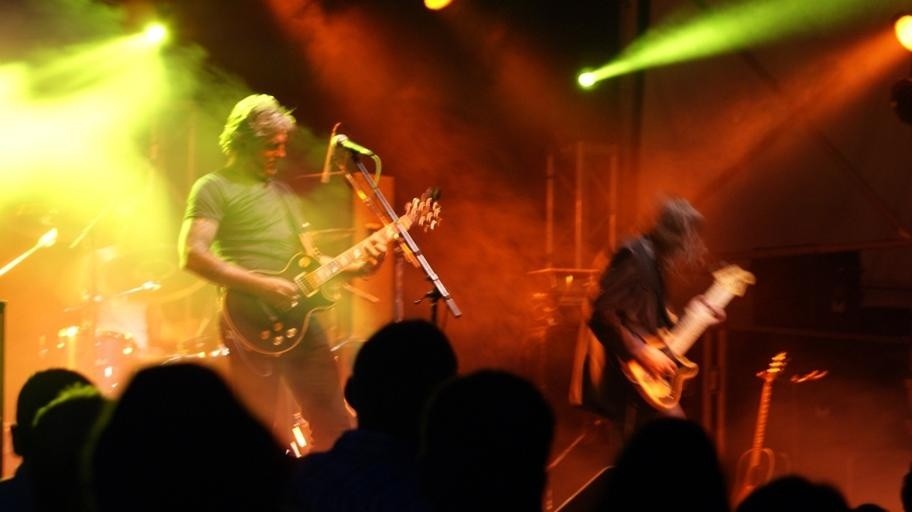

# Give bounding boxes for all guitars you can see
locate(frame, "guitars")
[220,188,440,359]
[730,352,787,509]
[621,267,753,411]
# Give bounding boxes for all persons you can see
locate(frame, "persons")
[177,93,388,460]
[568,198,726,440]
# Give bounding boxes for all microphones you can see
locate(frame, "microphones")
[335,134,373,159]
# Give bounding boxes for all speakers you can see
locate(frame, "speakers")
[522,267,599,392]
[544,435,613,511]
